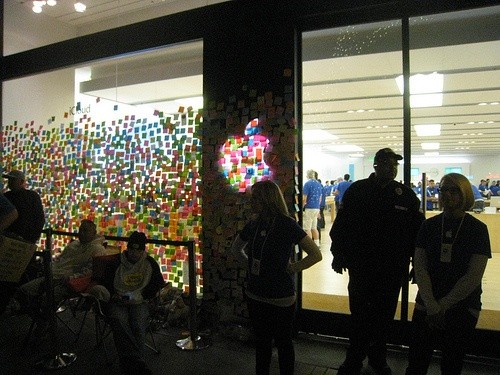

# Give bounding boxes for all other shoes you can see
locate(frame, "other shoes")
[338,359,364,375]
[369,360,393,374]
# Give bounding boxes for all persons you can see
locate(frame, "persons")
[230,180,322,375]
[1,169,45,316]
[1,191,19,238]
[329,148,425,375]
[16,220,109,355]
[404,173,492,375]
[101,231,165,375]
[301,169,499,252]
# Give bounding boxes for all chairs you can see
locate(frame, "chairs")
[93,254,160,371]
[20,239,108,351]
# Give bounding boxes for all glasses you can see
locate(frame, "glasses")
[437,188,461,195]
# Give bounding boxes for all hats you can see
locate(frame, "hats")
[374,148,403,165]
[128,231,146,250]
[2,170,25,180]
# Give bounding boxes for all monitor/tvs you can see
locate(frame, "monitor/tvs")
[444,168,462,176]
[411,168,419,178]
